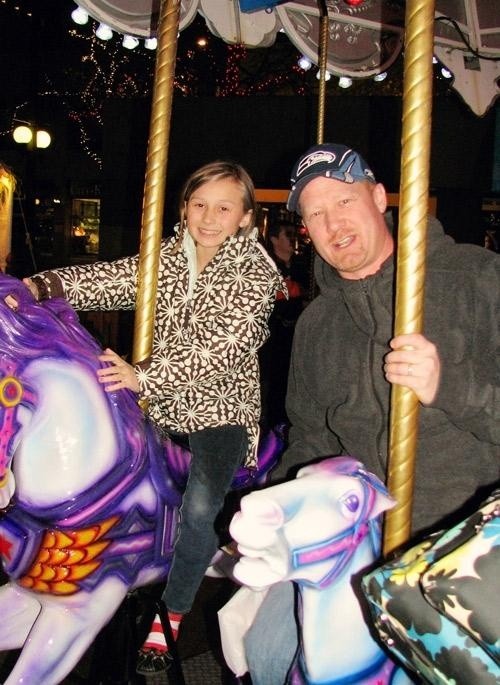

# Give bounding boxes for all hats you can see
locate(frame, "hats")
[286,144,378,215]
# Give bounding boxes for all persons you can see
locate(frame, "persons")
[260,143,500,560]
[1,158,291,678]
[258,218,322,430]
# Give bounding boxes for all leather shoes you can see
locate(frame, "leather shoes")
[134,640,175,676]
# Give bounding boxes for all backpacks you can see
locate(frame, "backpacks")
[350,484,500,685]
